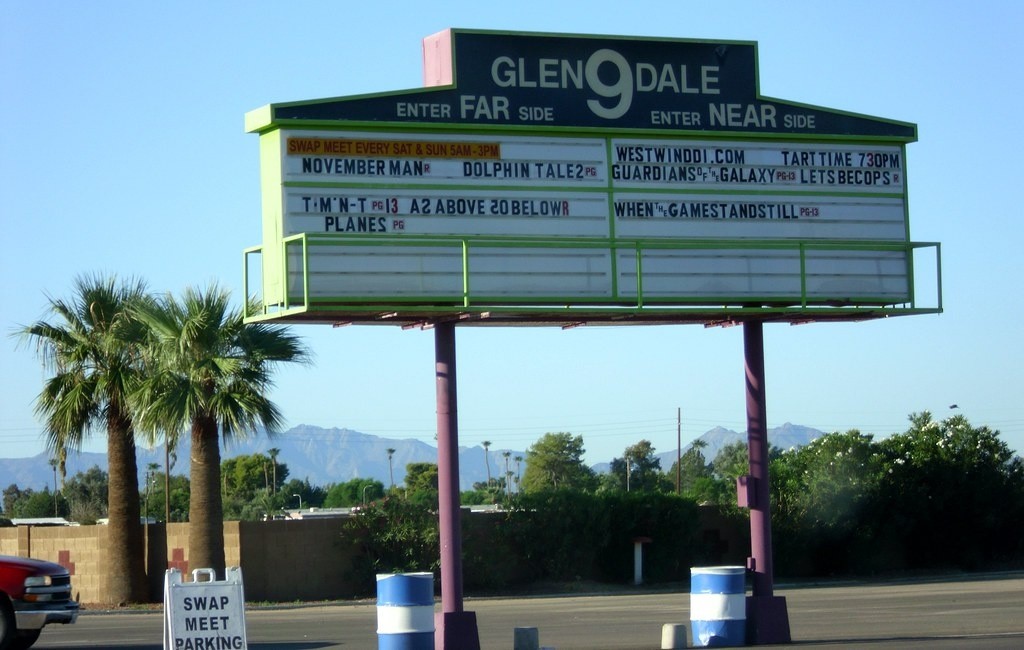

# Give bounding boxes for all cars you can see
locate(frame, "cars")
[0,553,80,650]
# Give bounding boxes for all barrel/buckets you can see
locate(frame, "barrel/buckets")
[375,572,435,650]
[689,566,745,648]
